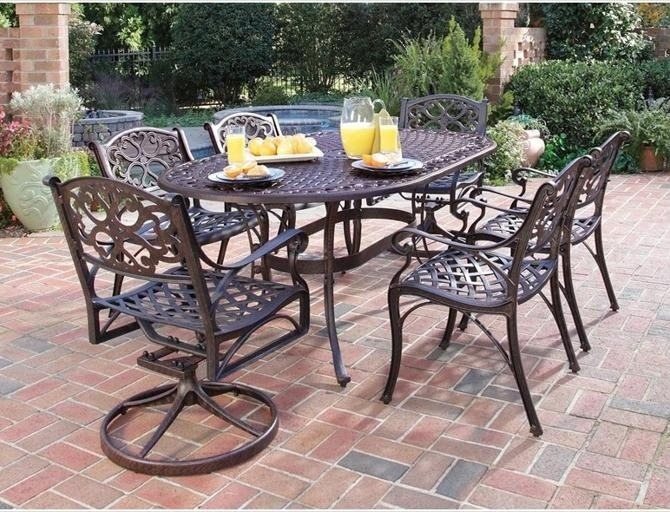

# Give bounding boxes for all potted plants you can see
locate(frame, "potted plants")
[487,115,551,167]
[591,92,670,172]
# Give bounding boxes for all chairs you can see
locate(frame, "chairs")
[204,113,352,274]
[386,95,490,257]
[379,154,593,436]
[89,126,270,343]
[43,174,310,476]
[457,131,633,352]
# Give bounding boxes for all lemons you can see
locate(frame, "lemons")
[223,159,269,178]
[363,152,387,167]
[248,133,313,156]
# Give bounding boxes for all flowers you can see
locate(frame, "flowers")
[0,80,85,160]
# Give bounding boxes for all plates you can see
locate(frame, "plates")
[209,169,287,185]
[247,147,324,164]
[351,158,423,174]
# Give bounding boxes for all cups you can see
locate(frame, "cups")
[225,125,246,164]
[379,117,398,152]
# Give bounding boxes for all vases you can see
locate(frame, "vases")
[0,150,91,232]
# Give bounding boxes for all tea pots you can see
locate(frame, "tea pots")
[340,97,387,159]
